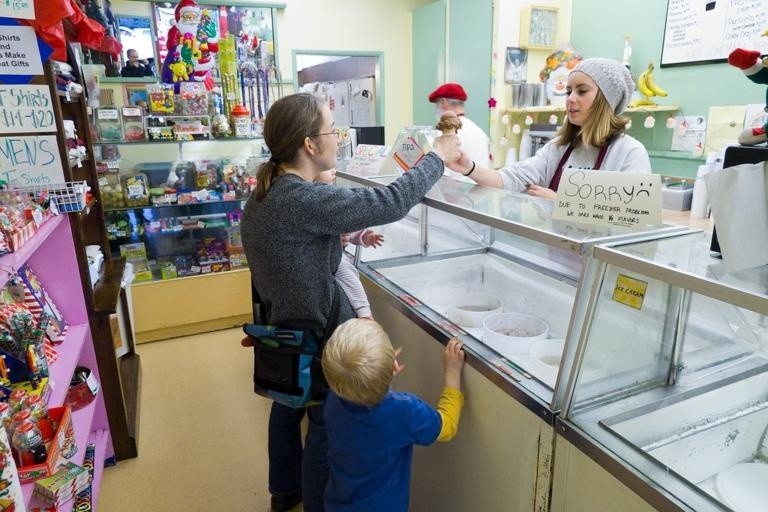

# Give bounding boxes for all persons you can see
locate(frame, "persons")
[120,49,149,77]
[320,317,465,510]
[312,164,385,324]
[446,57,651,202]
[167,1,215,82]
[239,92,465,509]
[430,82,492,178]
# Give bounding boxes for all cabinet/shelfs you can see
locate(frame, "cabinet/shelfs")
[329,78,376,127]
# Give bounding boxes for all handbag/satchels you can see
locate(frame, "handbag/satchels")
[243,321,320,410]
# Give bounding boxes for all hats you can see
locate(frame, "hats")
[428,83,466,102]
[568,58,634,115]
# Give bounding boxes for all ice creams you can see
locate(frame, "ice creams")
[436,111,463,135]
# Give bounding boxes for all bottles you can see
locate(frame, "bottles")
[211,105,252,138]
[0,388,55,468]
[388,124,440,179]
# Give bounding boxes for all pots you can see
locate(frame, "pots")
[512,82,545,109]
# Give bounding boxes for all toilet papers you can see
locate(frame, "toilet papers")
[703,145,768,274]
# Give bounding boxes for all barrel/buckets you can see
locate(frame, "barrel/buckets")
[531,338,565,375]
[448,294,502,324]
[481,312,547,350]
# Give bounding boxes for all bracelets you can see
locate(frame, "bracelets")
[464,160,476,178]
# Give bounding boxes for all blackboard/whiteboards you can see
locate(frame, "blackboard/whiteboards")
[659,0,768,68]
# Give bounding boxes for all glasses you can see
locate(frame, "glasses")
[310,129,341,139]
